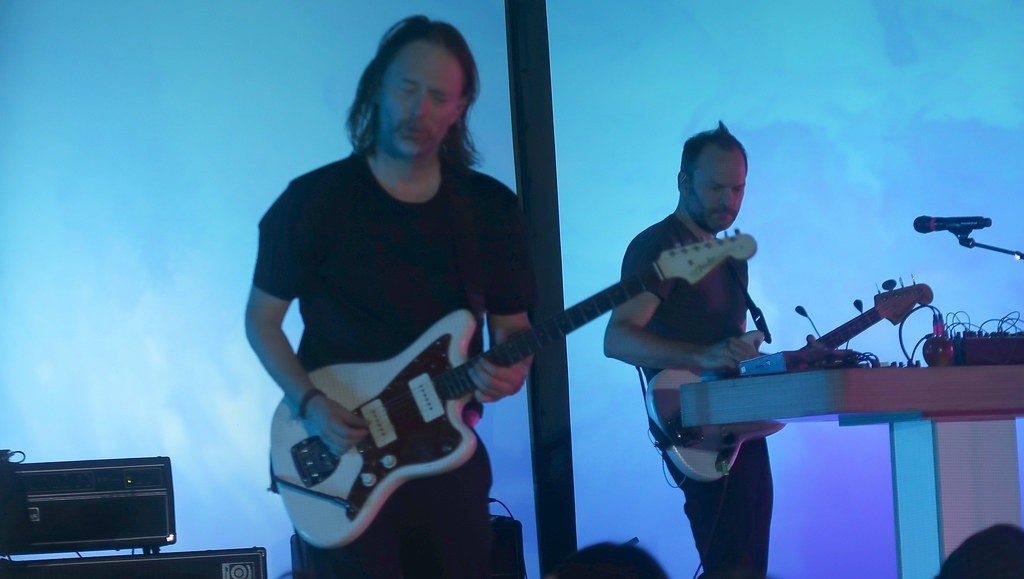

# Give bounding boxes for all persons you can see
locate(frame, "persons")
[604,121,826,579]
[935,525,1024,579]
[545,543,669,579]
[245,15,534,579]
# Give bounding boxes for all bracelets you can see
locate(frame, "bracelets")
[298,389,325,418]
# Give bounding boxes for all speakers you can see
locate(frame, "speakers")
[290,517,526,579]
[0,547,267,579]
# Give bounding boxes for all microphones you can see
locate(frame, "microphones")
[913,216,991,233]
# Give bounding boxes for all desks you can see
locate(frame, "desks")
[679,366,1024,579]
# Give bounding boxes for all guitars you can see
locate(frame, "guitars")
[646,274,934,481]
[267,229,759,549]
